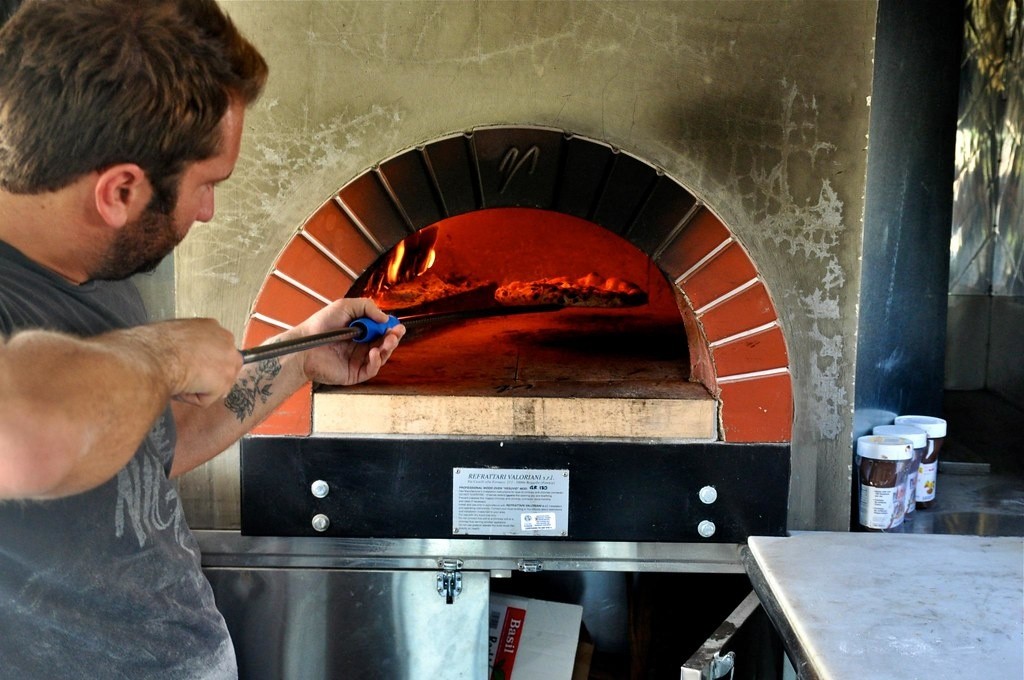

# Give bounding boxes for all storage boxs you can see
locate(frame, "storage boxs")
[487,591,595,680]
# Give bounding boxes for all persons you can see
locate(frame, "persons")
[0,0,406,680]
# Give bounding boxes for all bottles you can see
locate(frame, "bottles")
[856,435,913,530]
[872,424,928,514]
[894,415,947,509]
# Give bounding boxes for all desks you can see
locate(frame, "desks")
[740,538,1024,680]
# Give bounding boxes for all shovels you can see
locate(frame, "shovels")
[240,275,651,363]
